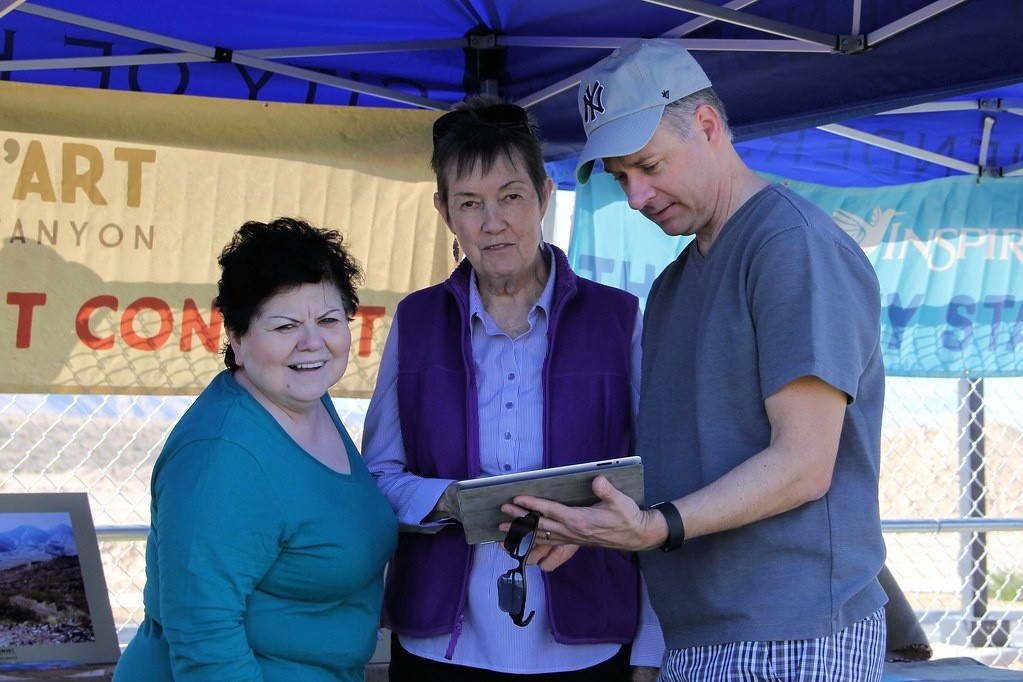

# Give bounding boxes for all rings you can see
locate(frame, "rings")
[545,531,551,542]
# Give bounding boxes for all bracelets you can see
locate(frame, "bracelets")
[648,501,685,553]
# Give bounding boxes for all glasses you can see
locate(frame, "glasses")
[432,103,529,148]
[497,510,543,626]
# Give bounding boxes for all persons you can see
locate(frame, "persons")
[362,93,666,682]
[110,216,400,682]
[497,37,889,682]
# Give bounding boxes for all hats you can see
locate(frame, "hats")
[573,37,713,184]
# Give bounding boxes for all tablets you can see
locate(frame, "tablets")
[452,456,646,546]
